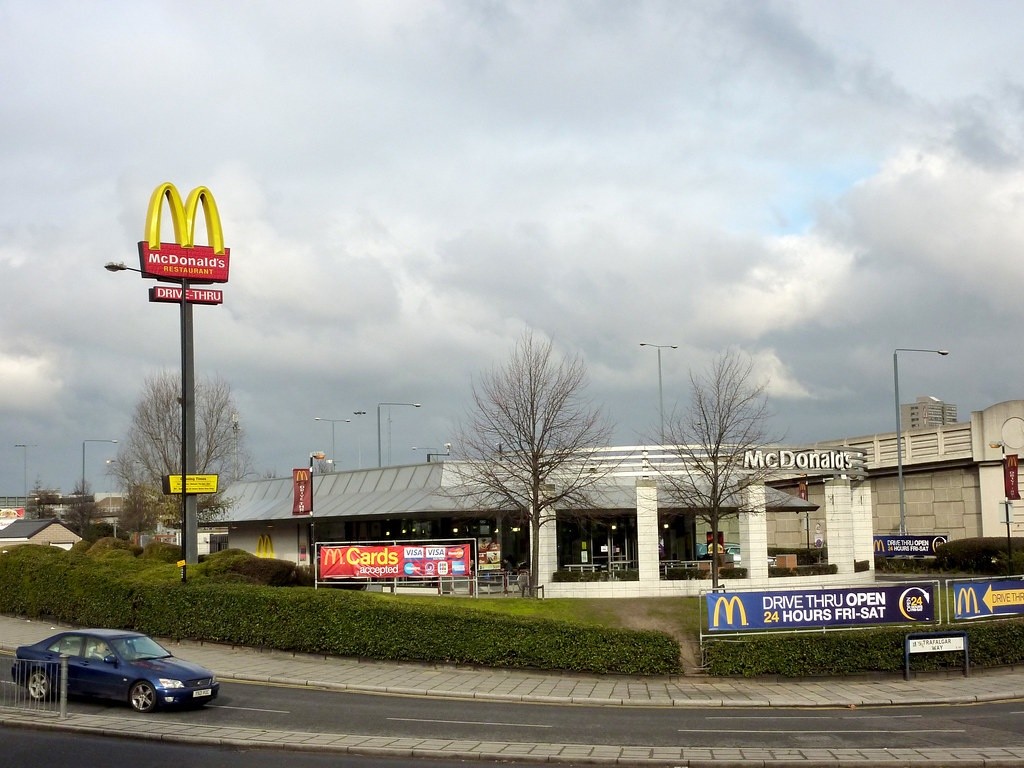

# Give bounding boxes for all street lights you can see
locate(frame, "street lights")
[640,343,678,462]
[82,440,118,500]
[990,440,1013,576]
[15,444,26,496]
[893,348,949,536]
[310,451,326,564]
[795,473,809,549]
[377,402,421,467]
[354,411,366,469]
[314,418,351,471]
[412,447,439,462]
[104,262,188,582]
[495,442,504,461]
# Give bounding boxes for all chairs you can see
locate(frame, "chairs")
[519,570,545,601]
[89,646,96,657]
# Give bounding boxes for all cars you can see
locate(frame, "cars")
[695,541,740,564]
[12,629,220,712]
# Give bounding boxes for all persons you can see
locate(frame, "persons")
[90,640,109,661]
